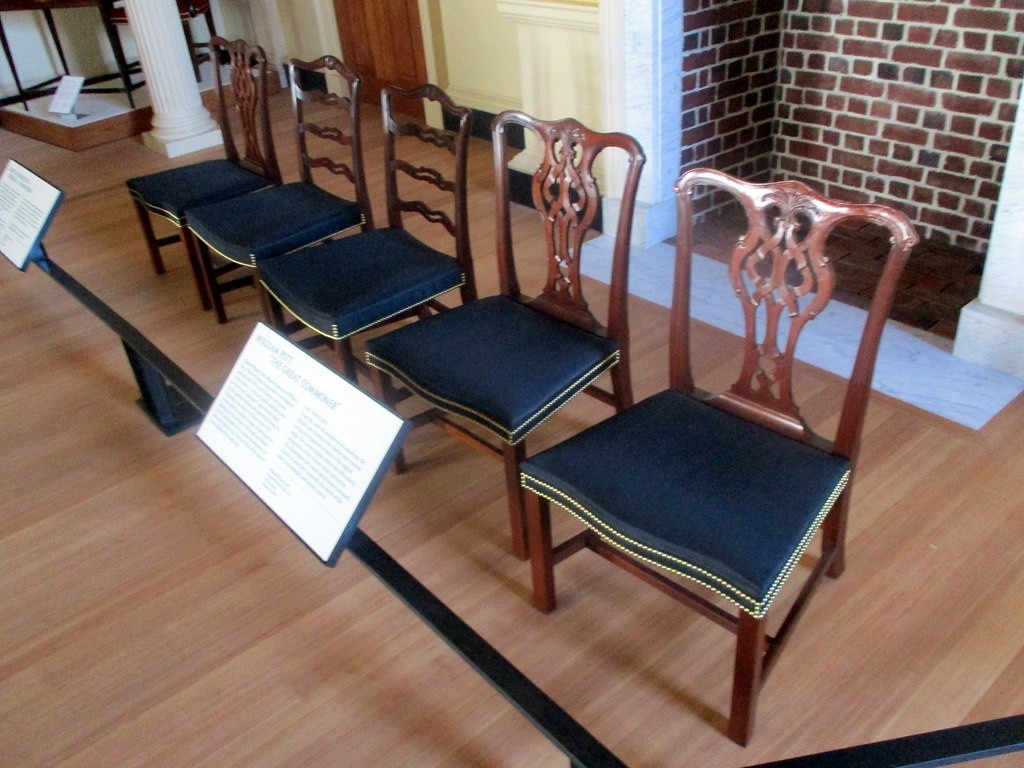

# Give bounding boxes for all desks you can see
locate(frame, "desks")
[0,0,148,110]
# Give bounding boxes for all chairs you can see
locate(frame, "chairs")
[518,168,920,750]
[185,55,374,340]
[109,0,216,83]
[366,110,646,562]
[255,80,479,389]
[125,36,284,310]
